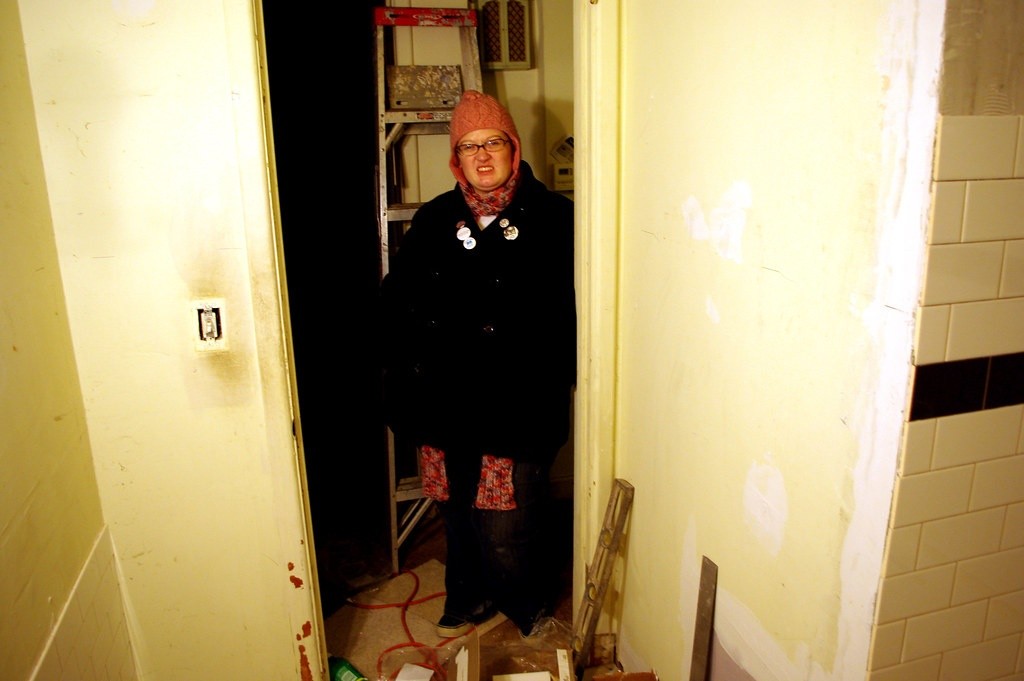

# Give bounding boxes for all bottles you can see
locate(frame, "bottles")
[328,653,370,681]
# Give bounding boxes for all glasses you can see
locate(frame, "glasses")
[455,138,509,156]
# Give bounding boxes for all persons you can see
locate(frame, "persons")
[371,90,576,638]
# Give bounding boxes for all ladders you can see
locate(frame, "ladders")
[359,5,488,574]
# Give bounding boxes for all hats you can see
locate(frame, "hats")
[449,90,521,196]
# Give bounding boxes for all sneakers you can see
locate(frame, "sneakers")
[517,607,547,644]
[437,600,492,638]
